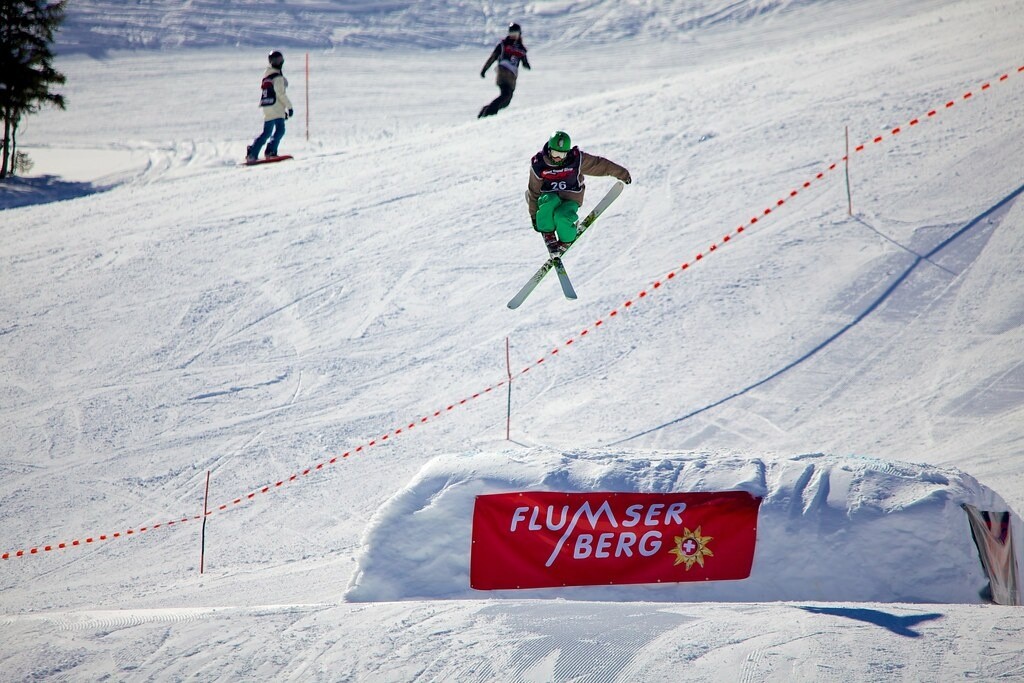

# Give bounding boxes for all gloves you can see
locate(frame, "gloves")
[624,175,632,184]
[523,63,530,69]
[532,219,539,232]
[288,109,293,116]
[285,112,288,119]
[481,70,486,77]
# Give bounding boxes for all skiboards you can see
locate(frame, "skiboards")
[525,189,578,301]
[506,181,624,310]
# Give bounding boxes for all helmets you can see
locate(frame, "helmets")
[268,51,282,57]
[548,131,571,152]
[508,22,521,33]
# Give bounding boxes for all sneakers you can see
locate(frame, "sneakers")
[542,232,558,253]
[557,240,571,252]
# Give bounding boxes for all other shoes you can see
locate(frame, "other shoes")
[477,105,494,118]
[245,146,257,161]
[265,143,279,158]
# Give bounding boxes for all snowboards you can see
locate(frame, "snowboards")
[239,154,294,165]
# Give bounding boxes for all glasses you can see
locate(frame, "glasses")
[550,149,568,159]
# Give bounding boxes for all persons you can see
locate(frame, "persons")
[528,131,632,251]
[478,22,531,118]
[246,51,293,161]
